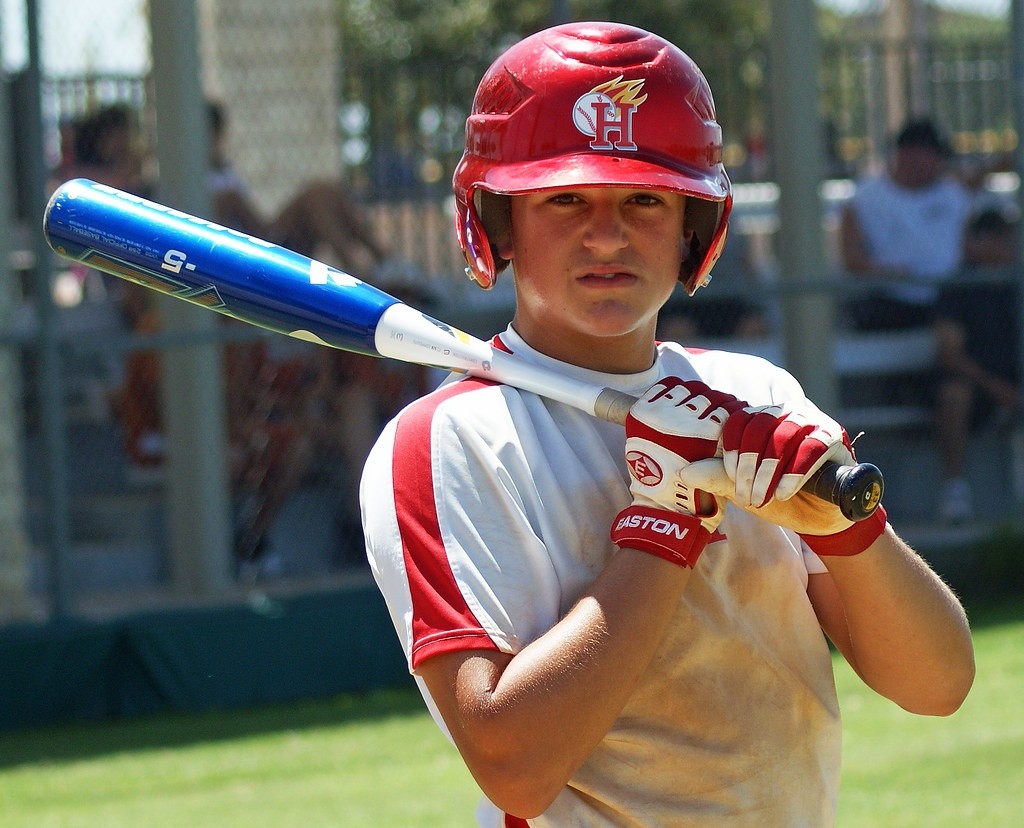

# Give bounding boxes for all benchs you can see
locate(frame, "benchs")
[651,196,950,433]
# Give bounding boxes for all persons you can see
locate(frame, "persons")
[662,114,1024,516]
[47,87,438,582]
[358,23,975,828]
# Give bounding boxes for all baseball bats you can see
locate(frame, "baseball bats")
[43,176,883,521]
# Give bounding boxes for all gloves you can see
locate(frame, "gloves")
[679,401,888,557]
[609,375,753,569]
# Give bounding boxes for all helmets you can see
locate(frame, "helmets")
[451,22,734,299]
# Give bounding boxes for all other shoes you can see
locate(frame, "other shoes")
[944,480,972,516]
